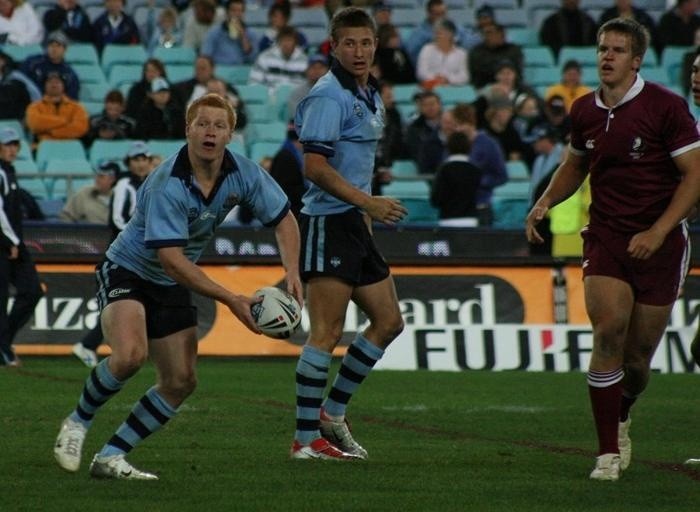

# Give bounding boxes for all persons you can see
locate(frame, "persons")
[524,17,700,481]
[53,92,304,480]
[0,0,700,263]
[683,55,700,476]
[0,129,46,368]
[287,7,410,464]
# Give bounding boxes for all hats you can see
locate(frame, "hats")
[149,76,171,94]
[548,95,567,117]
[308,53,329,67]
[0,127,21,145]
[128,141,153,159]
[93,160,120,178]
[48,31,67,46]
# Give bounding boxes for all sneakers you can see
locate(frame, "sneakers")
[0,344,21,368]
[53,417,87,473]
[590,453,621,482]
[90,453,160,481]
[288,406,367,462]
[618,412,631,470]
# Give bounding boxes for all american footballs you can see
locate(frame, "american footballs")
[252,287,302,338]
